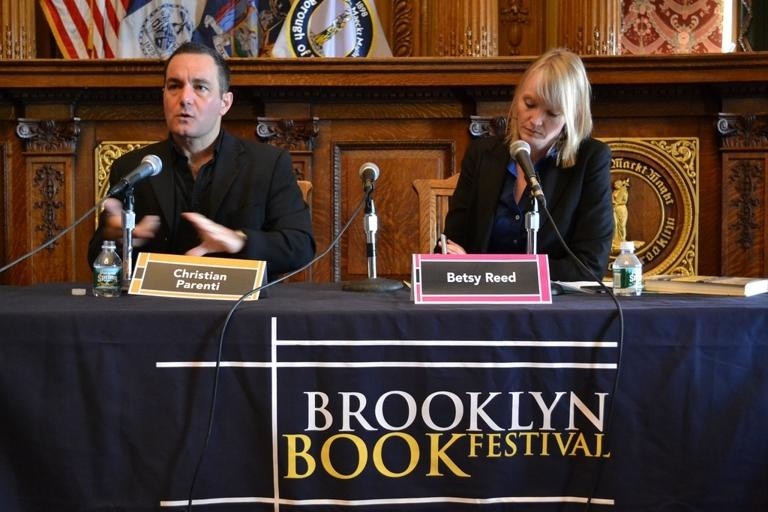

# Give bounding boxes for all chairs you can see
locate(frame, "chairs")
[414,177,462,256]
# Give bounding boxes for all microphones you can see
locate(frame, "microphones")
[358,161,381,194]
[508,138,547,209]
[108,152,163,198]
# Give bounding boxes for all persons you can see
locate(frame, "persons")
[89,43,317,285]
[612,180,629,241]
[434,48,615,281]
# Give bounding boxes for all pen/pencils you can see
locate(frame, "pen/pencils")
[441,234,447,254]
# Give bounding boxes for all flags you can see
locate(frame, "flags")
[38,1,393,58]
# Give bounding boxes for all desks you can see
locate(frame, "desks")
[1,280,768,510]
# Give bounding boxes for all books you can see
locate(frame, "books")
[644,275,768,297]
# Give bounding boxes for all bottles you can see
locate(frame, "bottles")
[612,242,643,297]
[91,242,122,298]
